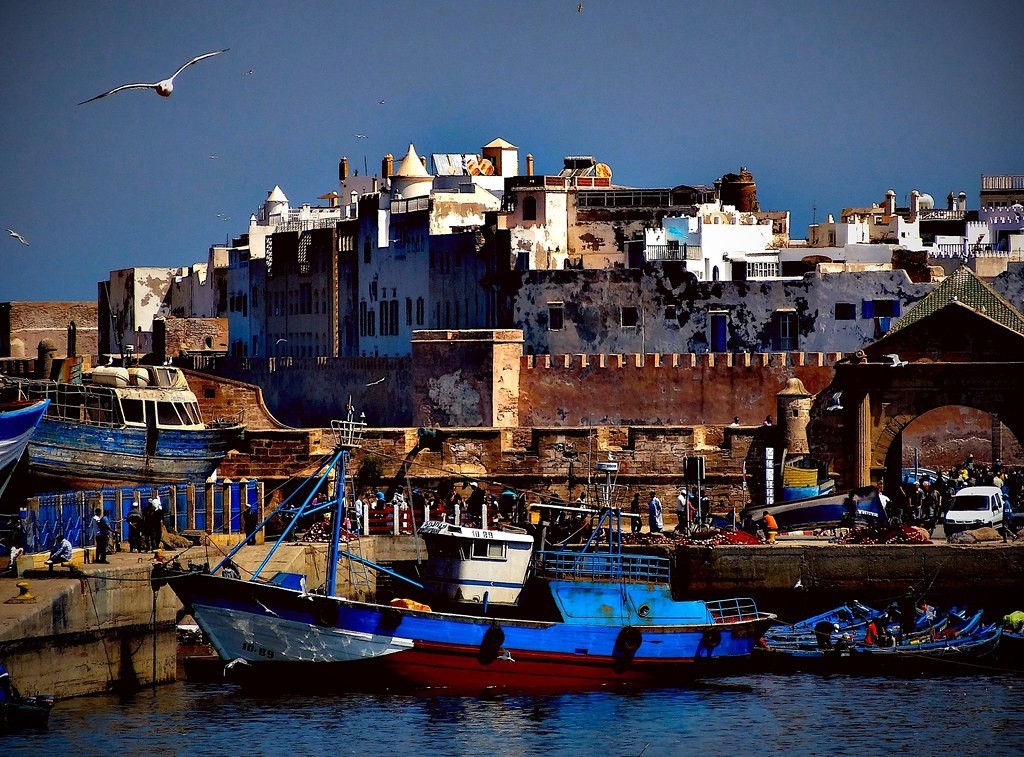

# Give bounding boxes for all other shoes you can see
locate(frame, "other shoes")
[95,559,109,564]
[44,560,54,564]
[1000,540,1007,543]
[1013,535,1017,542]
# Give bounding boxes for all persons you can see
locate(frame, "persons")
[675,486,709,532]
[761,511,778,537]
[243,503,257,545]
[630,493,642,533]
[44,535,73,571]
[814,617,896,650]
[648,492,664,532]
[222,559,241,580]
[311,480,519,536]
[876,459,1024,544]
[574,492,587,517]
[126,497,165,552]
[91,508,114,564]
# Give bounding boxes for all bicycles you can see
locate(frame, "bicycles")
[672,509,709,540]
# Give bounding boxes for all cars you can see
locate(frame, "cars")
[902,467,948,489]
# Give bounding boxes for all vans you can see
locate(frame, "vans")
[943,486,1005,536]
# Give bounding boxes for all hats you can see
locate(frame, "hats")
[246,503,252,508]
[470,481,477,487]
[1002,494,1009,498]
[413,489,419,494]
[132,502,138,507]
[681,489,687,494]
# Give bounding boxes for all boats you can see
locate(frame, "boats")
[154,390,781,692]
[740,464,888,530]
[0,344,248,506]
[754,596,1024,663]
